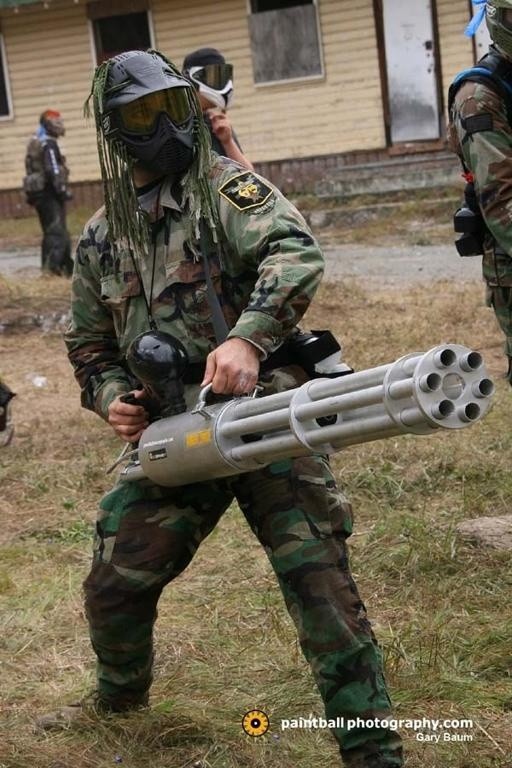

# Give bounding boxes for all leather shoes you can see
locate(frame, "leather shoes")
[30,689,100,735]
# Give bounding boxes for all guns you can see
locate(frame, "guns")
[106,328,494,488]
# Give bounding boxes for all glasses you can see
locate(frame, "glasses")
[104,88,197,141]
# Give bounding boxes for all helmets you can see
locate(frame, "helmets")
[94,50,200,183]
[185,47,235,111]
[486,0,512,55]
[40,110,65,138]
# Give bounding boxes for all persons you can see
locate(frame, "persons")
[23,110,75,279]
[447,0,512,389]
[181,47,256,177]
[68,46,407,766]
